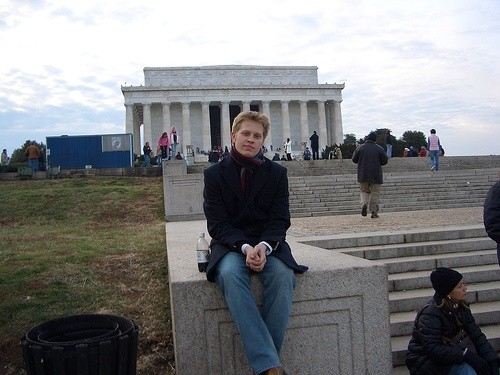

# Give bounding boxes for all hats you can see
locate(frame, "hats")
[431,267,463,296]
[368,134,377,141]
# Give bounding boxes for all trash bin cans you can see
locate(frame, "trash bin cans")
[22,314,139,375]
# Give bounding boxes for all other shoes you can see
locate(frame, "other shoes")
[431,165,436,171]
[371,213,380,217]
[361,203,367,217]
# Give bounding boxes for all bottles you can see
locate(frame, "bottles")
[196,233,210,273]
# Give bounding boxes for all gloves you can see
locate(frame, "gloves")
[463,348,490,375]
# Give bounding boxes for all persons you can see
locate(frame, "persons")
[208,146,229,165]
[323,145,342,160]
[141,142,152,168]
[262,138,311,161]
[24,142,40,171]
[427,129,441,171]
[1,149,10,165]
[352,133,388,218]
[404,144,444,157]
[309,131,319,160]
[157,126,182,166]
[405,267,500,375]
[203,110,309,375]
[483,181,500,267]
